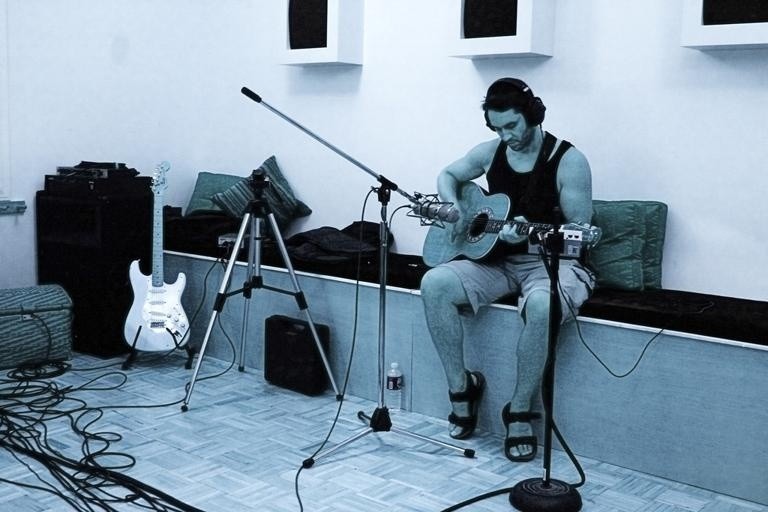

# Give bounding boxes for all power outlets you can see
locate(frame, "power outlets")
[1,199,27,217]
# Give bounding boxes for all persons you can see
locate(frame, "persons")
[421,77,593,462]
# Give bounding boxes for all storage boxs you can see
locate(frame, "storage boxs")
[1,284,75,370]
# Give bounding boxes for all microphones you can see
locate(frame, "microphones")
[410,203,460,222]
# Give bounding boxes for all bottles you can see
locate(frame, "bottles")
[387,362,403,415]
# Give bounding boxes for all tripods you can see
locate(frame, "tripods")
[175,168,343,412]
[242,86,476,469]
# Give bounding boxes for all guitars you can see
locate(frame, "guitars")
[422,179,601,268]
[122,161,192,352]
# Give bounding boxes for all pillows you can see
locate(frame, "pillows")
[185,172,242,215]
[639,200,668,291]
[591,200,645,288]
[213,155,313,243]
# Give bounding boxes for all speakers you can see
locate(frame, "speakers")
[263,314,329,398]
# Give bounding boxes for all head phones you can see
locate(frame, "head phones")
[484,81,548,132]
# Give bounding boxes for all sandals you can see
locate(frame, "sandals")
[448,367,485,440]
[502,402,541,463]
